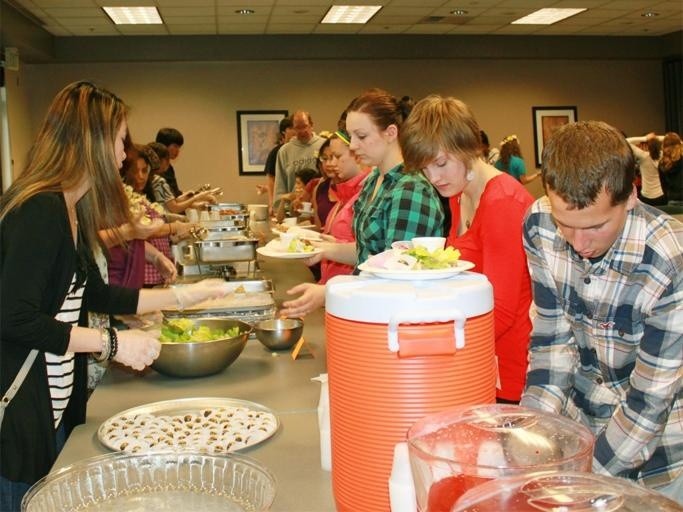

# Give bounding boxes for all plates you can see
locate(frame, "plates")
[270,226,325,242]
[98,396,279,463]
[278,222,316,229]
[357,258,475,281]
[256,246,322,260]
[148,279,274,314]
[297,209,315,216]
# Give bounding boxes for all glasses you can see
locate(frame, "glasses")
[319,155,333,163]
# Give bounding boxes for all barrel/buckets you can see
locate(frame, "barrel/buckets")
[324,271,496,512]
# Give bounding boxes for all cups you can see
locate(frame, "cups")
[301,201,312,210]
[283,216,298,226]
[412,236,447,257]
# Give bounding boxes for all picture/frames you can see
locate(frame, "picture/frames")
[235,110,289,176]
[532,106,579,169]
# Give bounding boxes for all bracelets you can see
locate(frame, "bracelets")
[108,325,118,363]
[97,326,110,365]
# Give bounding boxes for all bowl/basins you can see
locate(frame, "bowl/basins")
[208,199,241,212]
[254,318,304,350]
[249,220,270,236]
[20,447,279,512]
[221,213,248,219]
[248,204,269,221]
[193,234,258,264]
[189,220,247,232]
[137,318,254,380]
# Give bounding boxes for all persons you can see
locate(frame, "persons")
[98,202,176,286]
[318,128,372,285]
[158,129,216,214]
[315,137,340,233]
[626,135,669,206]
[122,150,201,292]
[291,154,326,221]
[145,142,214,212]
[0,80,225,507]
[265,118,299,216]
[519,120,683,490]
[397,95,536,405]
[659,132,682,201]
[277,167,318,224]
[494,134,542,184]
[274,110,325,206]
[279,90,445,320]
